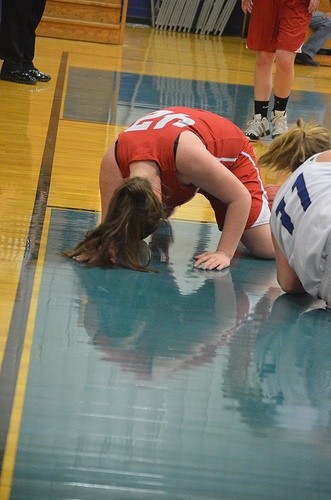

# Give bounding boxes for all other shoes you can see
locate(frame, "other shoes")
[294,53,320,67]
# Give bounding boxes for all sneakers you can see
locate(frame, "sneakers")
[244,114,270,142]
[0,69,37,85]
[263,184,282,202]
[270,110,288,140]
[24,68,51,82]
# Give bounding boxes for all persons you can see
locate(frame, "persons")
[63,106,276,272]
[240,0,320,143]
[294,10,331,68]
[0,0,51,85]
[258,116,331,311]
